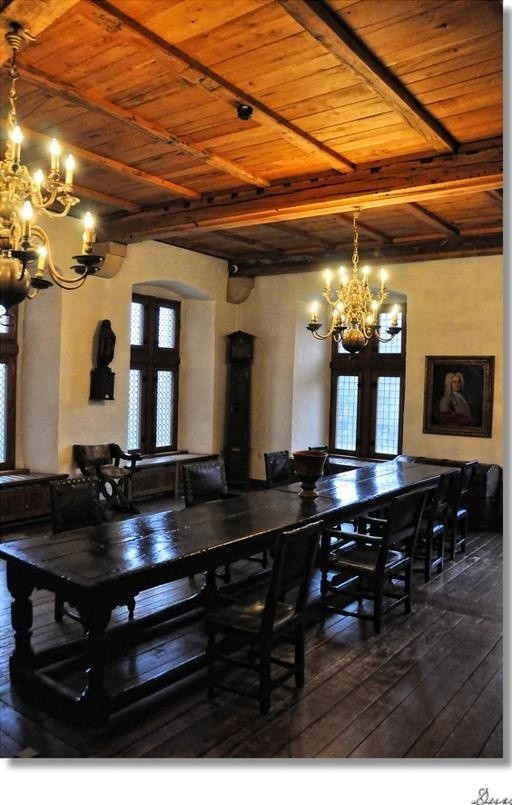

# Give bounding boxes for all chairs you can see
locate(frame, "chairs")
[72,443,143,514]
[205,519,324,710]
[262,449,343,540]
[181,454,270,581]
[319,486,429,624]
[415,469,460,582]
[432,460,479,559]
[45,478,135,633]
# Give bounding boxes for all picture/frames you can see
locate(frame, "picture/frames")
[422,355,496,439]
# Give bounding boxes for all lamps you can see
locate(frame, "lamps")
[308,210,404,361]
[0,22,104,327]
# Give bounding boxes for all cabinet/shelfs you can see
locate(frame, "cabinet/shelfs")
[0,468,69,532]
[126,449,211,503]
